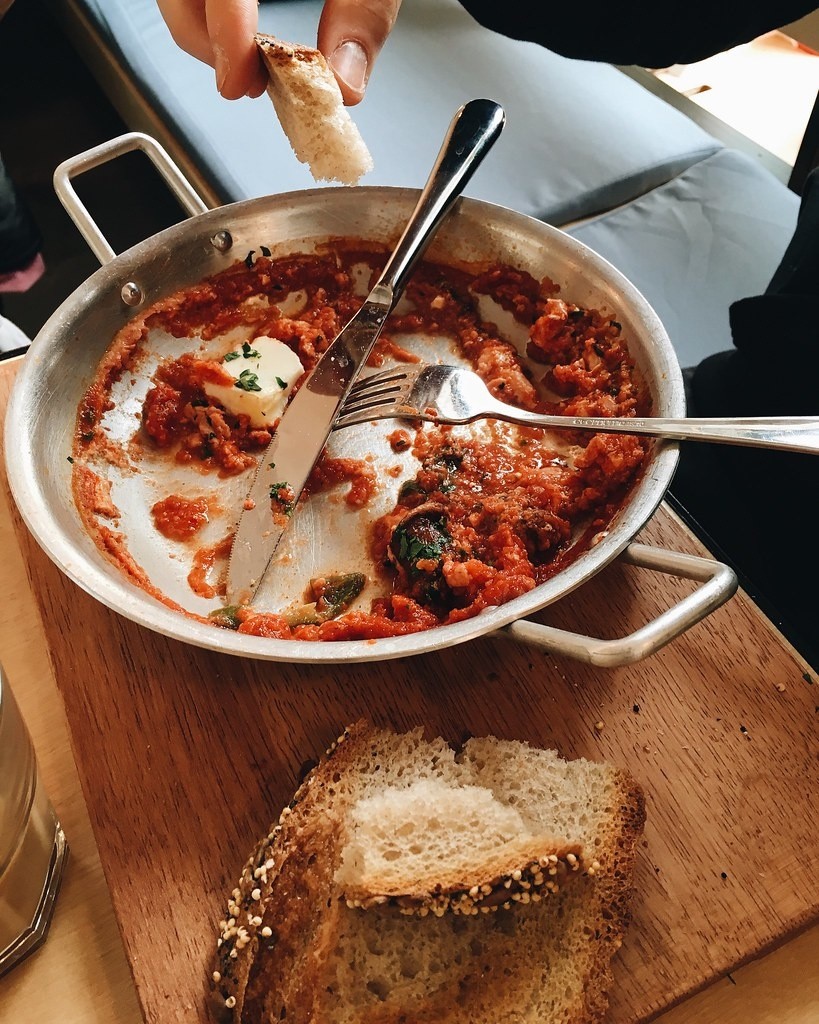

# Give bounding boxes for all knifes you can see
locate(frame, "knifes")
[225,97,506,607]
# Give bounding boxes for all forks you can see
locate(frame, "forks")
[331,363,819,456]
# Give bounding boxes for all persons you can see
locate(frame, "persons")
[157,0,819,670]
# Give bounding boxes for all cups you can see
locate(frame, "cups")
[1,672,72,978]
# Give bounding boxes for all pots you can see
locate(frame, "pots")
[2,129,738,668]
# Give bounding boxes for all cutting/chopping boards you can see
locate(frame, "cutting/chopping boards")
[0,355,819,1024]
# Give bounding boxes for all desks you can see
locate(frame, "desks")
[0,354,819,1024]
